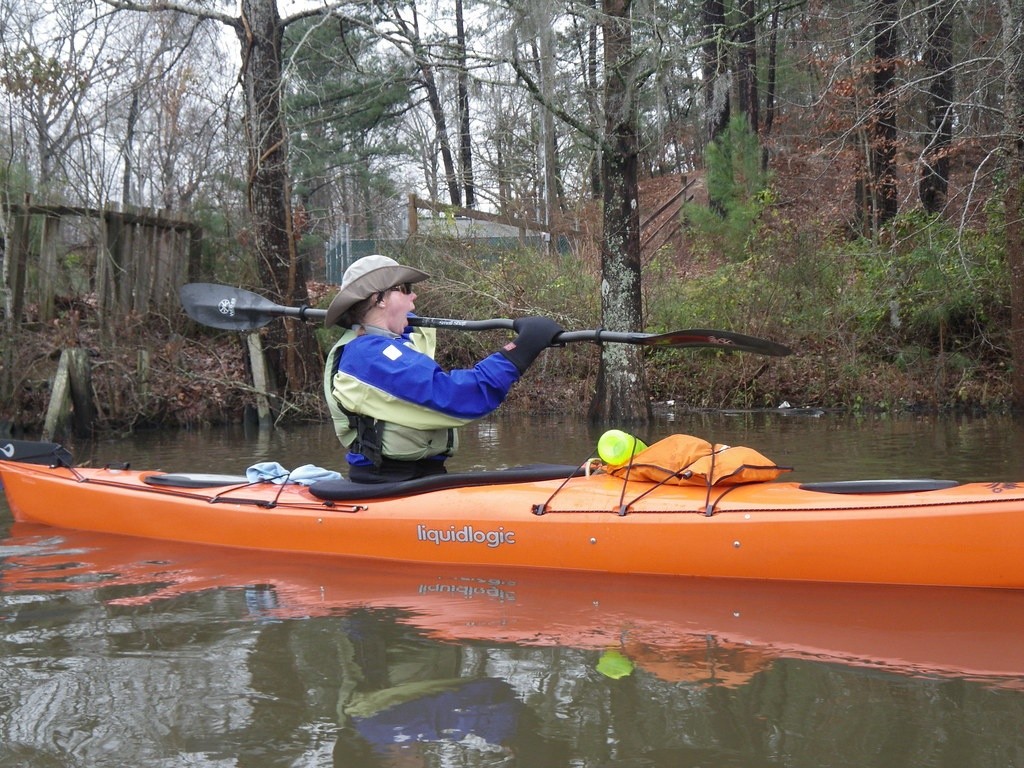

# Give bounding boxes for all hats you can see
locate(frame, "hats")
[325,255,431,329]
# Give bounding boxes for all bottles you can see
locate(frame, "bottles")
[597,429,648,465]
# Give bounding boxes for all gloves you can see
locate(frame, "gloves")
[499,317,568,375]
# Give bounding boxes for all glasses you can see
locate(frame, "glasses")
[391,283,411,294]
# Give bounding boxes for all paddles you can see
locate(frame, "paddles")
[176,281,794,361]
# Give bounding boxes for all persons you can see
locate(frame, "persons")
[334,613,567,768]
[322,255,566,484]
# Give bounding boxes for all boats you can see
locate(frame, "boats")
[0,429,1024,590]
